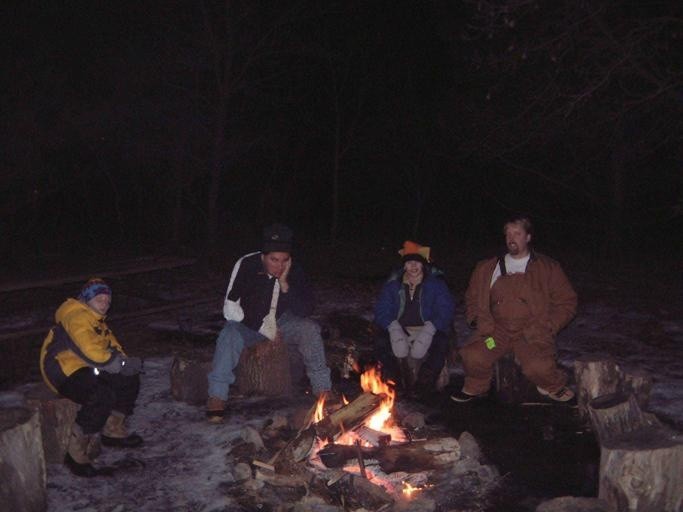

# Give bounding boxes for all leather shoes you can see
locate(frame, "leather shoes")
[409,321,436,360]
[387,320,413,358]
[103,352,128,375]
[117,356,144,375]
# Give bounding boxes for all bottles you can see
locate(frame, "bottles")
[260,227,296,258]
[81,276,113,302]
[398,240,431,266]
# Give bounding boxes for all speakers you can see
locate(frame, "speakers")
[101,410,143,449]
[408,364,437,407]
[64,429,97,477]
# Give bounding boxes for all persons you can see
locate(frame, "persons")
[41,279,145,477]
[376,240,453,403]
[206,222,337,424]
[449,212,575,403]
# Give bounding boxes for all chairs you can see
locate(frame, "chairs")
[450,382,488,402]
[537,374,575,403]
[206,391,225,423]
[312,386,340,410]
